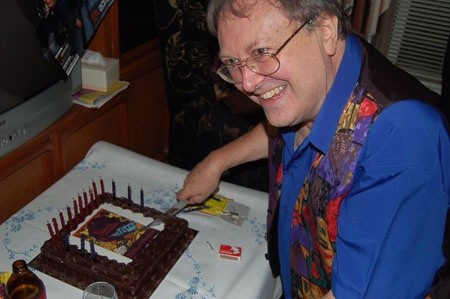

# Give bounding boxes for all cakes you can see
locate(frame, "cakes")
[40,193,189,297]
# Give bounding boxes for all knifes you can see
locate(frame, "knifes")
[146,199,189,226]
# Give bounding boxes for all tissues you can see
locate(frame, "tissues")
[81,49,120,92]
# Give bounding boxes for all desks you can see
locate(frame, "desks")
[0,138,284,299]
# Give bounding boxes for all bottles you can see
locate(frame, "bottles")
[5,260,46,299]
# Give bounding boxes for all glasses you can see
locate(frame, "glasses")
[217,18,312,84]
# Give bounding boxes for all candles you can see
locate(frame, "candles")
[46,176,145,262]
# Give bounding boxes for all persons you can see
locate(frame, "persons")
[176,0,450,299]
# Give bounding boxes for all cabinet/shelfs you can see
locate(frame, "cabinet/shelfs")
[0,0,169,226]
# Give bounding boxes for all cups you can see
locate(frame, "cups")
[83,281,119,299]
